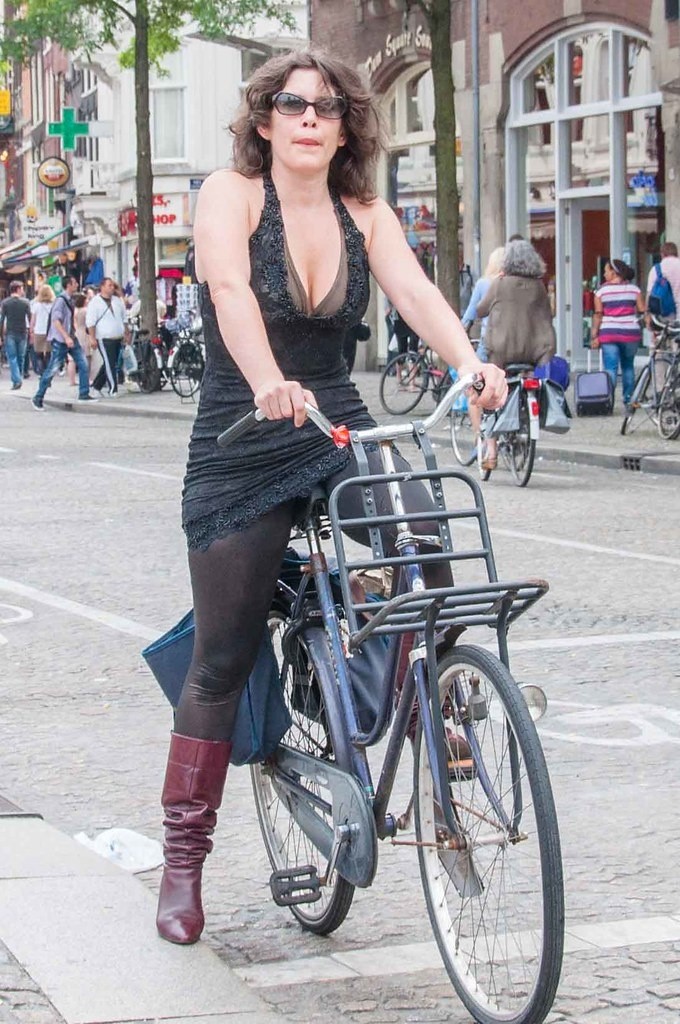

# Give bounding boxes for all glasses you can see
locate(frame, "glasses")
[269,91,349,121]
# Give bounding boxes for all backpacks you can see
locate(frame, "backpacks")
[649,262,677,318]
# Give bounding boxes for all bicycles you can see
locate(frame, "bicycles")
[216,369,577,1024]
[619,312,679,445]
[118,309,207,405]
[450,361,545,487]
[377,340,483,416]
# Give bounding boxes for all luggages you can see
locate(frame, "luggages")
[574,344,616,418]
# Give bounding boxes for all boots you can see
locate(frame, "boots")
[395,623,478,759]
[155,731,230,947]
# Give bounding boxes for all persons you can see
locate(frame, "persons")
[457,237,679,469]
[393,308,420,393]
[1,273,163,409]
[155,56,510,948]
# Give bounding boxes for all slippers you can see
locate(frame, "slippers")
[409,387,424,393]
[68,382,80,387]
[396,379,406,385]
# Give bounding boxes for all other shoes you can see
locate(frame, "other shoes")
[109,393,122,399]
[11,380,24,391]
[90,389,105,398]
[481,458,500,471]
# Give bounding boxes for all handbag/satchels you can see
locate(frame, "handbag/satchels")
[387,333,401,353]
[479,383,522,435]
[140,597,292,768]
[536,377,572,436]
[288,577,400,738]
[121,345,138,375]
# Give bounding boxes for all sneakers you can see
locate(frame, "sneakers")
[32,398,46,412]
[77,394,101,402]
